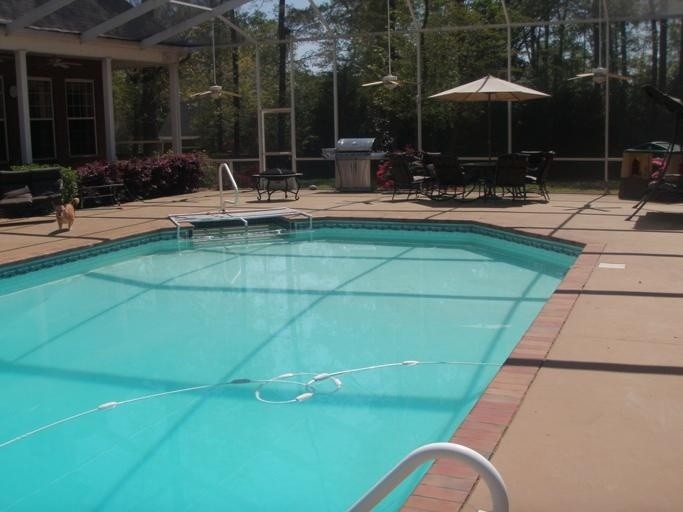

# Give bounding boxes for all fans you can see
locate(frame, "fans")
[361,1,418,90]
[567,1,633,87]
[190,19,242,100]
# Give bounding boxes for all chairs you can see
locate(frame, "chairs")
[390,150,556,204]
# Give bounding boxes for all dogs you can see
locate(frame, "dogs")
[52,197,81,233]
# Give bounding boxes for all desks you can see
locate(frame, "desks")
[251,172,305,202]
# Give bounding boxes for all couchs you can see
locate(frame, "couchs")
[0,166,63,217]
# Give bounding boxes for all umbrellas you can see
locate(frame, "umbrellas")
[425,73,552,195]
